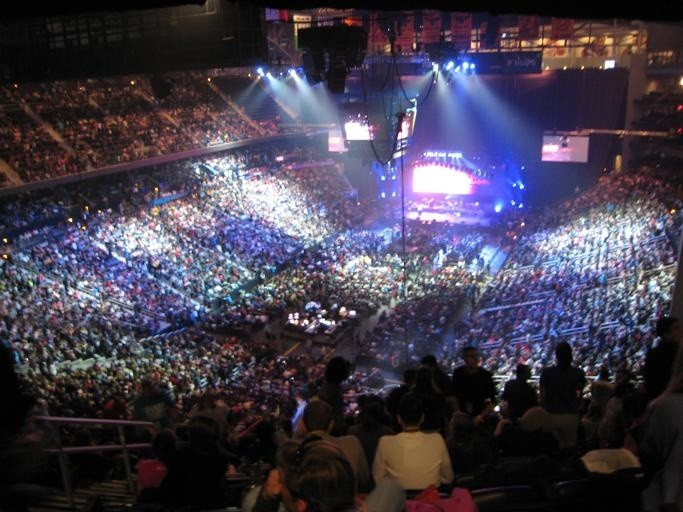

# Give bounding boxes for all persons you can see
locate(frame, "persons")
[0,67,681,511]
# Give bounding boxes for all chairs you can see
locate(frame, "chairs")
[127,443,682,512]
